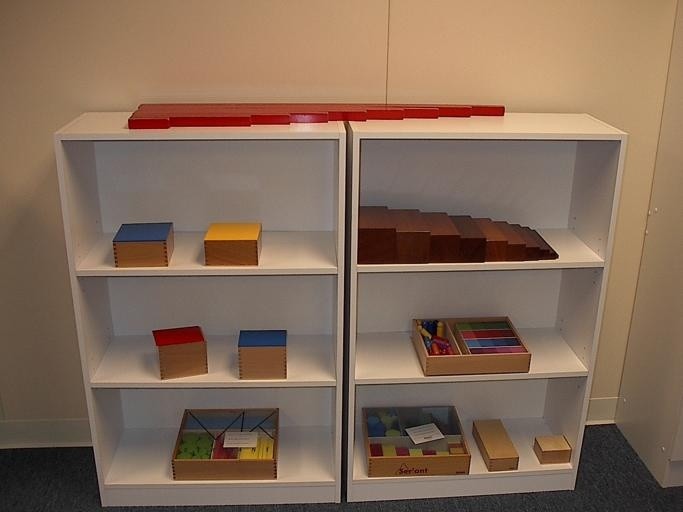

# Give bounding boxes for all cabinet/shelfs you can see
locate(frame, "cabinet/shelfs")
[54,113,347,508]
[346,113,629,502]
